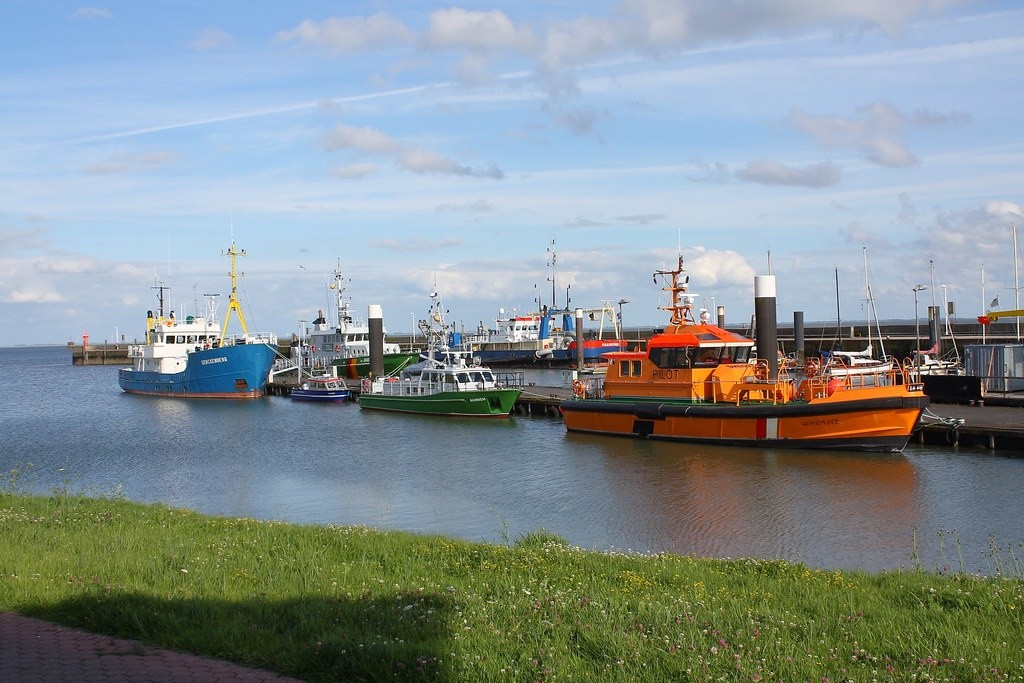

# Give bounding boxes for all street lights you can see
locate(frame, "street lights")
[913,284,928,419]
[617,298,630,352]
[409,312,416,343]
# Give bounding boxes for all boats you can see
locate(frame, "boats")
[289,226,1024,401]
[357,275,525,418]
[289,372,352,403]
[117,214,280,398]
[556,228,932,454]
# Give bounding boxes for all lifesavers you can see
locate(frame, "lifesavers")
[335,344,340,352]
[804,361,817,378]
[752,361,769,380]
[572,382,583,394]
